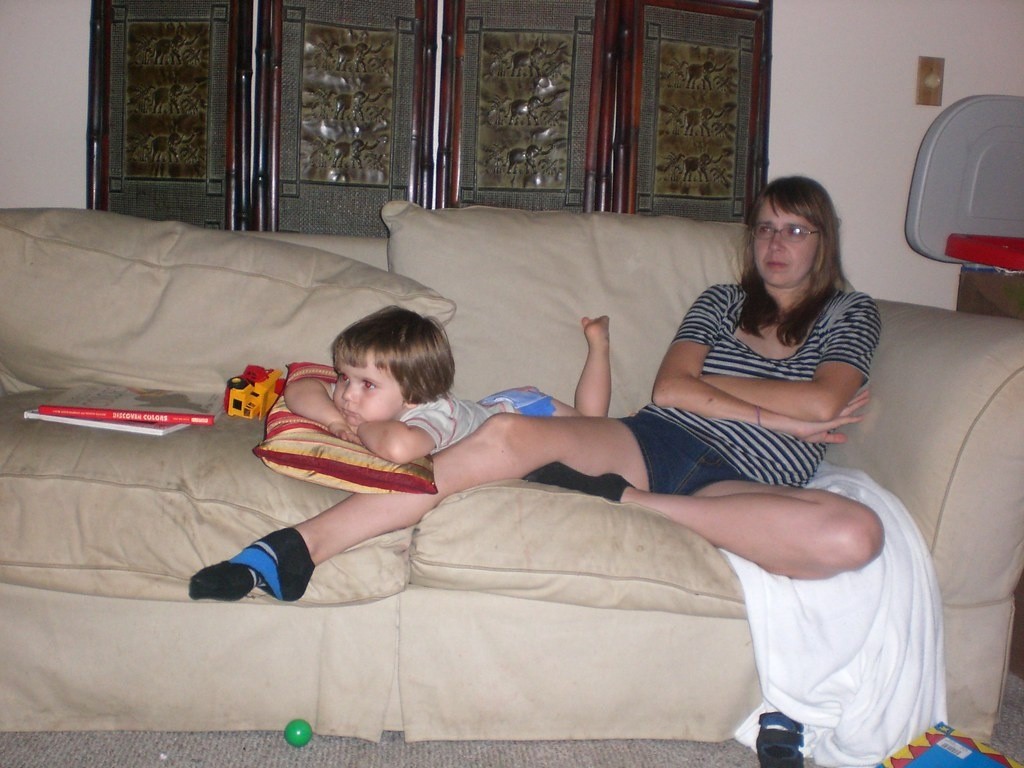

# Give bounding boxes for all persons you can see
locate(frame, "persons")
[189,176,882,602]
[284,306,611,464]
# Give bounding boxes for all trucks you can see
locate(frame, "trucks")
[224,365,285,423]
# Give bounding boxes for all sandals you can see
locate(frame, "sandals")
[757,711,807,768]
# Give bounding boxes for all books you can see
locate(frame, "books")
[24,385,224,436]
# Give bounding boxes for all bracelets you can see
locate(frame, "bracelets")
[756,406,761,426]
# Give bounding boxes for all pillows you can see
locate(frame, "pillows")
[382,199,754,418]
[0,208,458,412]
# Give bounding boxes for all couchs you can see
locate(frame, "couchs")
[0,231,1023,744]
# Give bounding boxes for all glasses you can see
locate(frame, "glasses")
[751,225,821,243]
